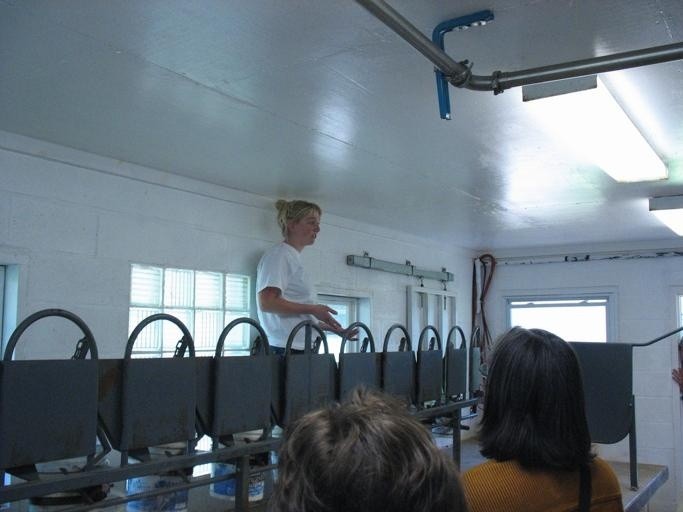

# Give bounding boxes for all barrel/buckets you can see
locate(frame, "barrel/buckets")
[10,446,110,512]
[124,441,191,511]
[415,396,483,450]
[211,429,265,502]
[270,425,287,484]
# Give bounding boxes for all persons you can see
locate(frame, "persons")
[267,383,467,512]
[255,198,360,356]
[457,326,625,512]
